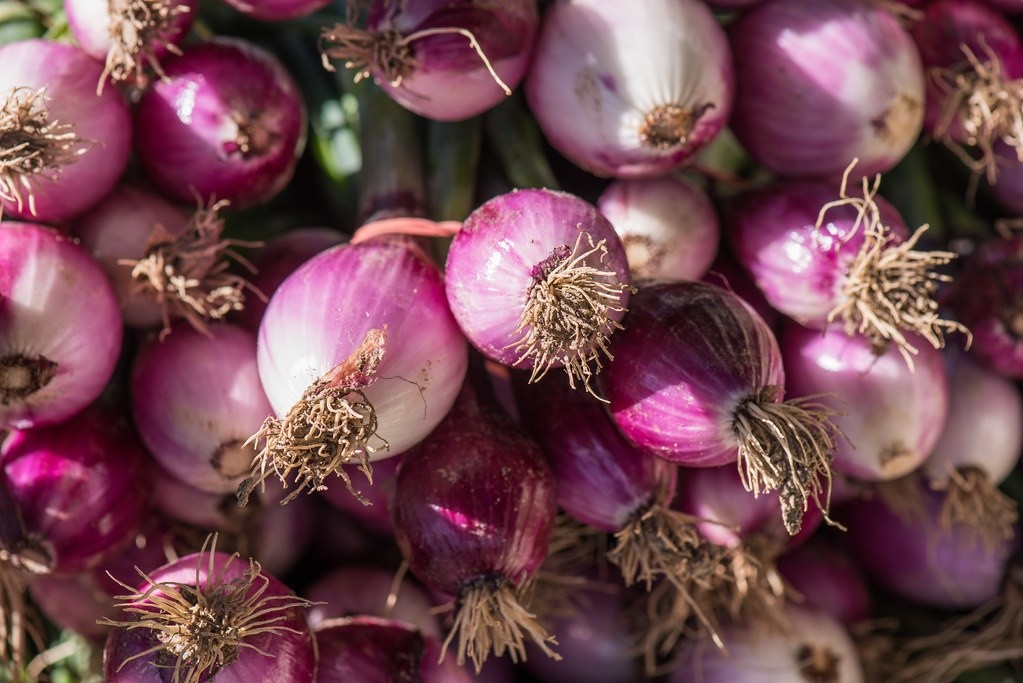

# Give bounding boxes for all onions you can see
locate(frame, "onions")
[0,0,1023,683]
